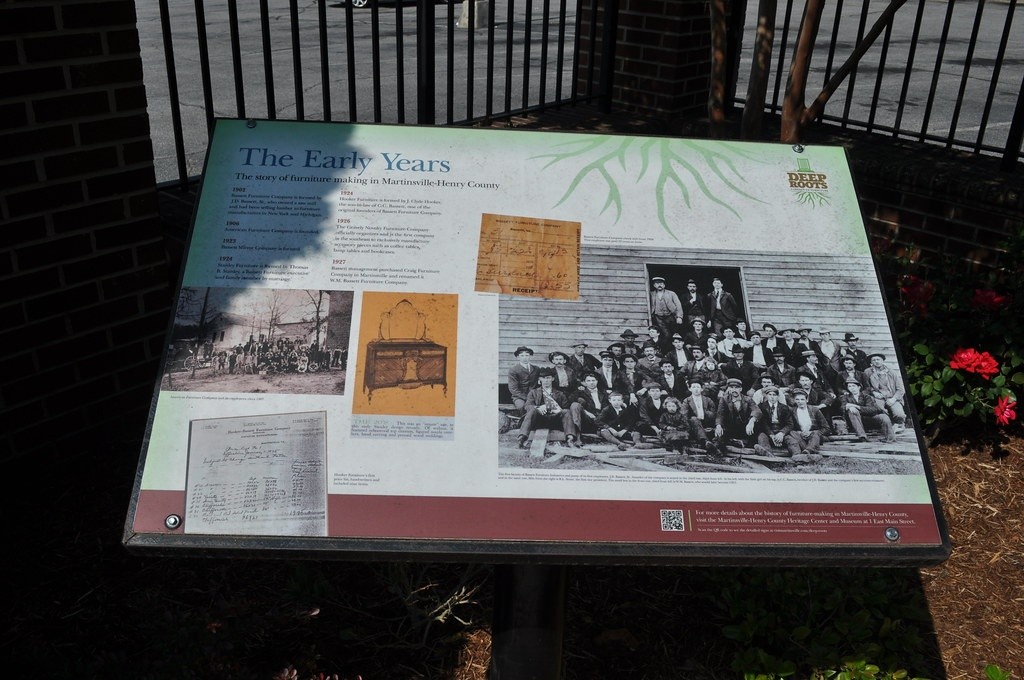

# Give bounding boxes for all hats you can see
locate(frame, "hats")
[513,345,533,357]
[672,333,682,341]
[817,326,831,333]
[620,329,639,338]
[720,323,735,333]
[778,327,795,336]
[747,332,761,341]
[549,352,569,364]
[642,342,655,350]
[621,351,638,363]
[570,339,588,348]
[584,371,600,380]
[689,343,703,350]
[795,325,812,333]
[599,351,614,357]
[763,322,777,334]
[841,332,859,341]
[607,343,624,351]
[866,350,885,360]
[538,368,554,376]
[690,316,705,325]
[647,350,863,394]
[652,277,666,283]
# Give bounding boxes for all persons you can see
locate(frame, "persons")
[507,276,907,456]
[175,333,348,376]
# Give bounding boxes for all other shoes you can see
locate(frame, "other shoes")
[512,412,903,463]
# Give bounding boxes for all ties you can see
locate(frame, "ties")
[716,292,719,299]
